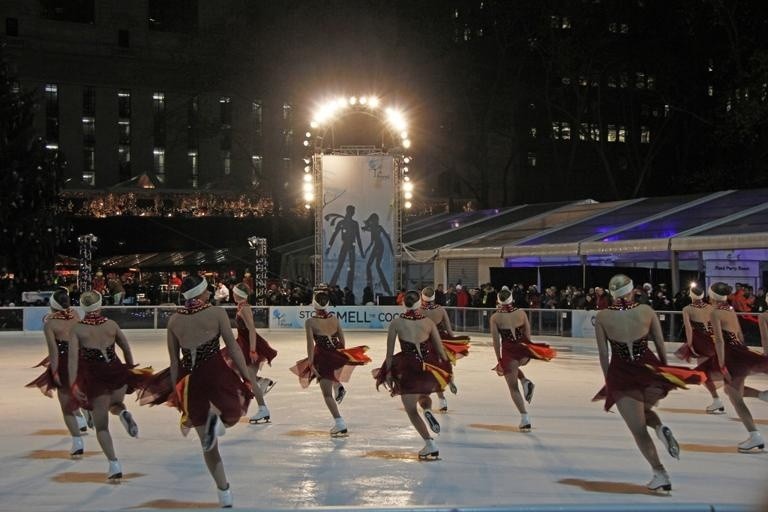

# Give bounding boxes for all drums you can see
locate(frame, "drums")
[159,284,168,293]
[170,285,180,294]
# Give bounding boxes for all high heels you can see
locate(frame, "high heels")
[522,380,533,400]
[334,384,344,401]
[217,484,232,506]
[419,439,439,456]
[73,409,89,435]
[758,391,768,404]
[449,380,457,395]
[422,409,440,434]
[706,397,724,410]
[203,413,226,453]
[739,431,764,449]
[646,467,672,490]
[106,461,122,479]
[249,406,270,421]
[657,425,679,458]
[119,410,138,437]
[330,417,347,434]
[68,437,83,456]
[438,397,448,410]
[260,379,272,394]
[519,412,531,428]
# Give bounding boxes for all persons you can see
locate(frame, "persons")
[372,289,459,458]
[137,273,257,508]
[757,291,768,359]
[361,211,395,298]
[21,286,91,457]
[674,282,726,414]
[1,265,373,332]
[416,286,471,412]
[66,289,140,480]
[294,291,369,435]
[709,284,768,454]
[216,281,278,422]
[435,282,768,344]
[489,284,556,428]
[324,204,365,290]
[588,273,709,496]
